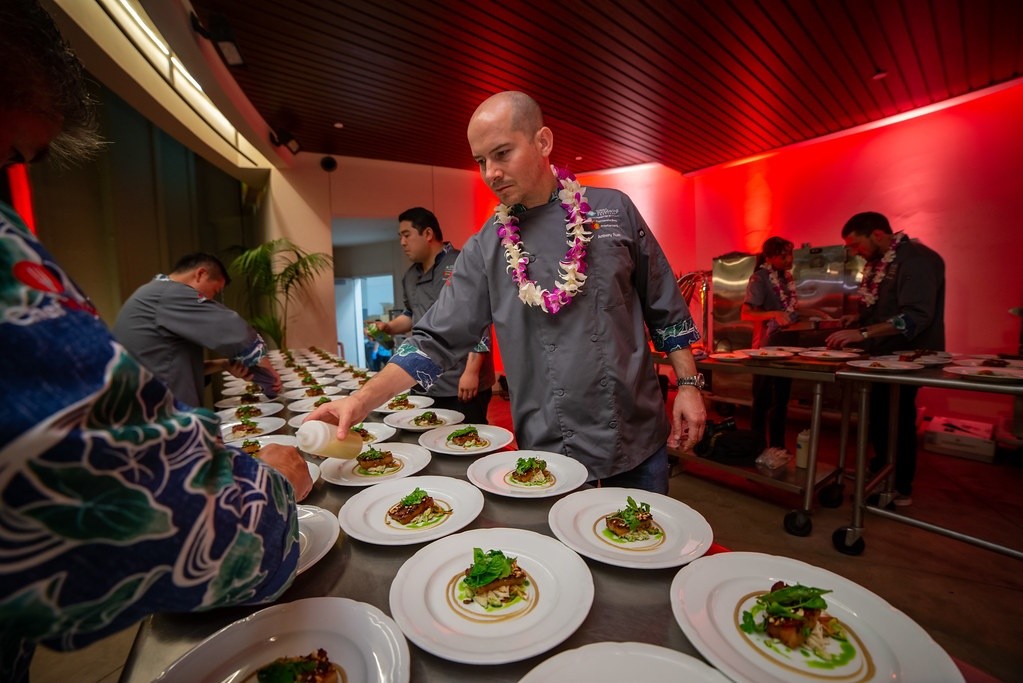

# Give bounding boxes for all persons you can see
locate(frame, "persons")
[741,237,800,450]
[302,91,708,496]
[824,211,946,504]
[364,206,496,424]
[0,0,314,683]
[112,253,283,408]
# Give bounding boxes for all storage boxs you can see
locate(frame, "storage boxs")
[924,416,997,460]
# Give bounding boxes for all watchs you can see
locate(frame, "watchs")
[677,373,705,390]
[859,328,868,338]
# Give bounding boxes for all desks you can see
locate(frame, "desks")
[116,370,1002,683]
[646,354,859,533]
[836,364,1023,562]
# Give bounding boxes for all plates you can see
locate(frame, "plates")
[339,476,485,545]
[389,527,595,665]
[149,596,410,683]
[952,358,1023,369]
[467,450,588,498]
[295,503,339,575]
[548,486,714,569]
[709,345,963,370]
[942,366,1023,381]
[205,345,589,487]
[515,641,734,683]
[670,552,966,683]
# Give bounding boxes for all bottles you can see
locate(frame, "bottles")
[362,321,395,349]
[295,420,362,460]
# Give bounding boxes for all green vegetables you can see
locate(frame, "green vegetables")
[463,547,513,588]
[739,581,834,635]
[236,347,478,460]
[610,496,650,532]
[403,487,429,505]
[516,457,547,475]
[257,656,317,683]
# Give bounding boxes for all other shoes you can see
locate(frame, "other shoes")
[861,490,911,506]
[846,464,887,480]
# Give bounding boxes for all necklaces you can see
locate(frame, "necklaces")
[493,165,595,314]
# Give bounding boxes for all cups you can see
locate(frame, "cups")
[796,434,811,468]
